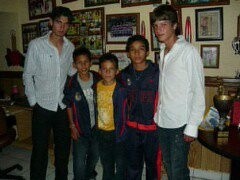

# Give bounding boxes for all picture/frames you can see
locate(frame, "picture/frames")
[28,0,56,19]
[201,44,220,68]
[109,50,131,74]
[39,19,52,36]
[195,7,224,40]
[149,8,182,51]
[106,12,140,44]
[64,7,106,64]
[62,0,230,8]
[21,22,39,53]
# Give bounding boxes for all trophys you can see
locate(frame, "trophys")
[213,76,234,146]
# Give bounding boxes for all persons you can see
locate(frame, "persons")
[114,35,159,180]
[152,4,205,180]
[22,0,230,69]
[91,52,127,180]
[23,6,78,180]
[201,15,209,35]
[62,48,103,180]
[208,50,213,66]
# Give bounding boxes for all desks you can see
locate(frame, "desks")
[9,107,32,141]
[198,125,240,180]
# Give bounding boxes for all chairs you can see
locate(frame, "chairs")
[0,107,24,180]
